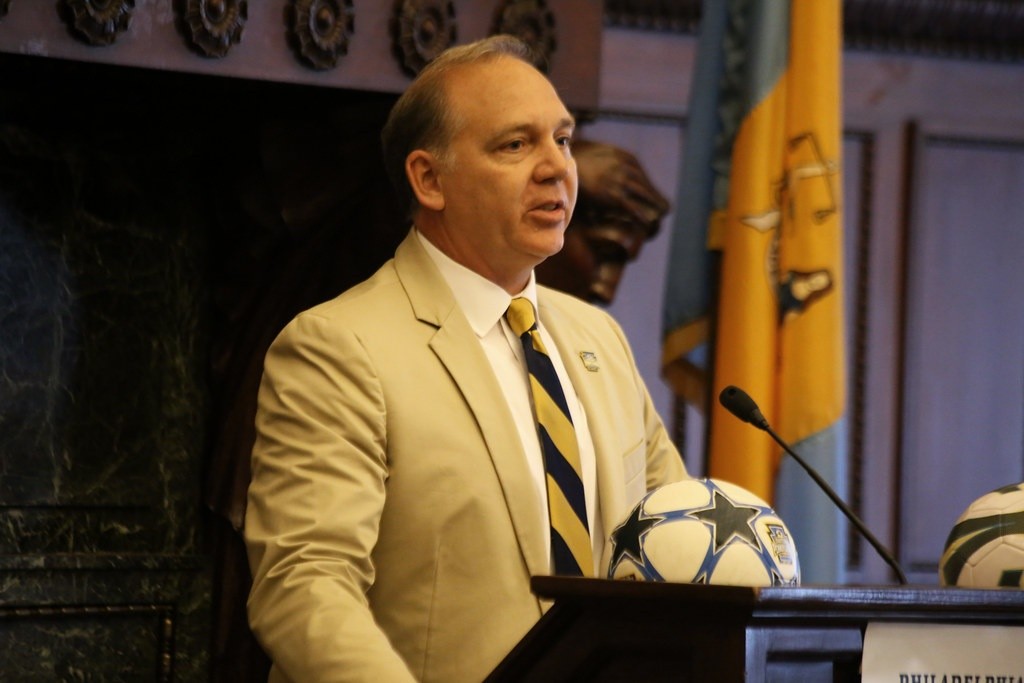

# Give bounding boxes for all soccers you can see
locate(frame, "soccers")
[937,481,1024,588]
[600,476,803,588]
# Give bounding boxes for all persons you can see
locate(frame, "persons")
[535,139,670,308]
[244,36,690,683]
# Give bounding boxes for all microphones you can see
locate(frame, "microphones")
[718,385,924,589]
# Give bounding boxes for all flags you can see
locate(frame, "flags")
[659,0,851,586]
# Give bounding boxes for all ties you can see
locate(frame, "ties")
[504,297,596,579]
